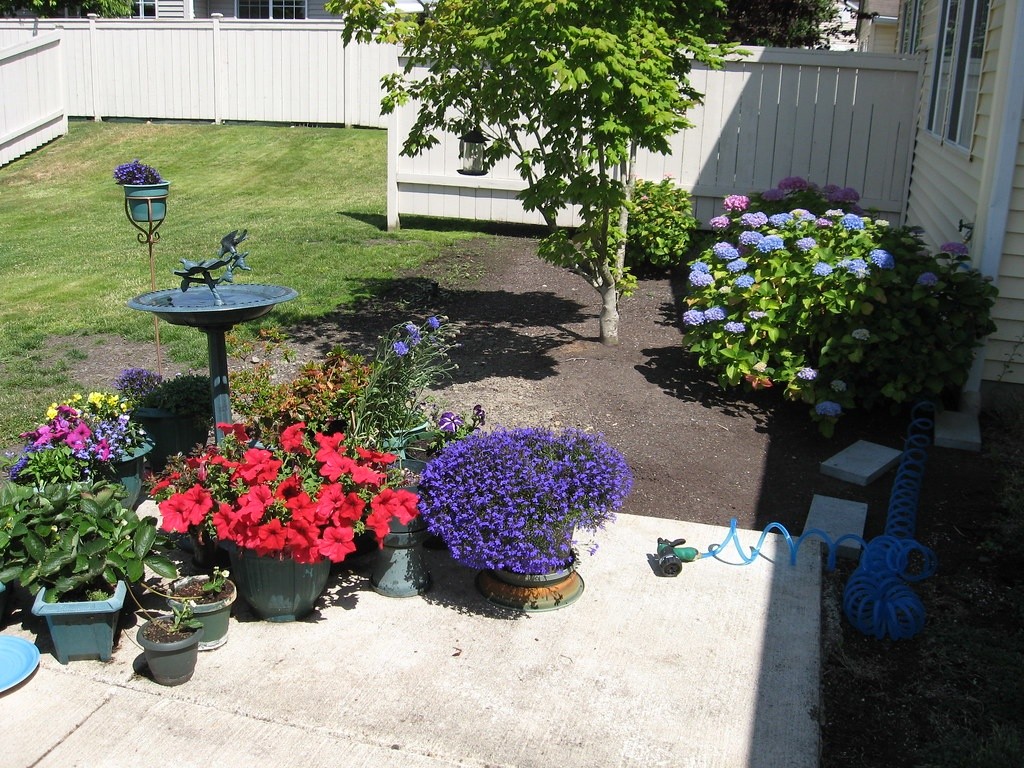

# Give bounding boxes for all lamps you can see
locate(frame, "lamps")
[457,125,491,176]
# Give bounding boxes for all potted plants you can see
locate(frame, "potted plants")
[137,601,205,688]
[133,376,214,470]
[1,481,203,663]
[360,423,433,597]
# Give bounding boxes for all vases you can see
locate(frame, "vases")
[507,523,577,573]
[403,432,440,463]
[227,544,332,624]
[113,438,156,506]
[165,574,236,651]
[125,181,171,222]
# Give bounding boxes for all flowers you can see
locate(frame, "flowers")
[113,160,164,185]
[143,423,422,566]
[415,426,633,574]
[1,389,152,485]
[204,567,230,596]
[426,405,486,457]
[344,314,466,433]
[114,368,163,405]
